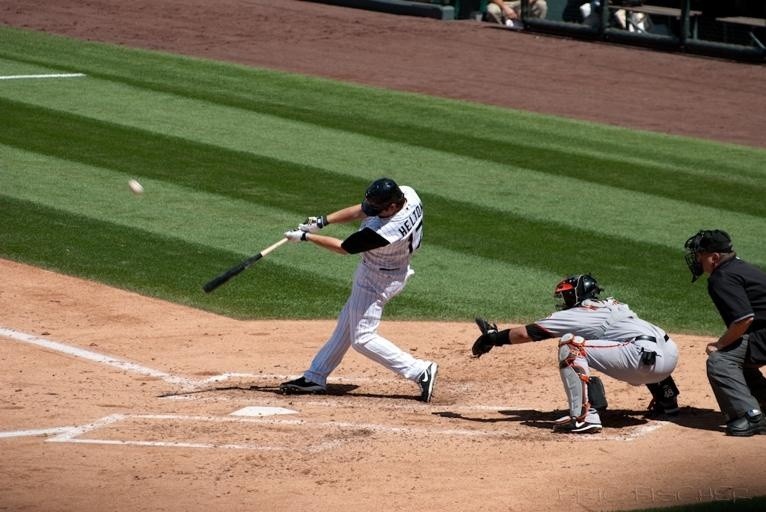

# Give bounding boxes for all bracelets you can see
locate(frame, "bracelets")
[323,214,328,226]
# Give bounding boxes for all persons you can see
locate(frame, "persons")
[472,273,680,433]
[685,229,765,437]
[483,0,547,28]
[279,177,438,402]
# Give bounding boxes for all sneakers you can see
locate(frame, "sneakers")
[280,376,327,395]
[726,410,766,437]
[416,362,438,403]
[553,408,602,433]
[653,397,680,413]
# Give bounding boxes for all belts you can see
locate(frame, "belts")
[626,334,669,343]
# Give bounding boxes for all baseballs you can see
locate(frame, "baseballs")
[129,181,142,195]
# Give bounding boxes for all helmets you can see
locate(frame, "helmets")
[361,179,397,217]
[684,229,734,283]
[554,273,604,311]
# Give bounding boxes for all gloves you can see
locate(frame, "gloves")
[283,215,324,241]
[641,347,657,365]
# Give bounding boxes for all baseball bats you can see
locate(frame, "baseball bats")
[204,217,318,293]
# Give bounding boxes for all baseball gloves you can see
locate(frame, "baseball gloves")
[472,317,500,356]
